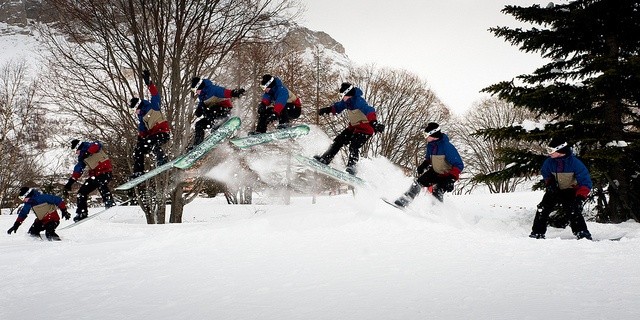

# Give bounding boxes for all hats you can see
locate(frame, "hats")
[339,83,356,96]
[71,139,83,150]
[261,74,275,88]
[547,137,570,154]
[191,77,205,90]
[129,97,143,108]
[17,187,33,198]
[425,122,441,138]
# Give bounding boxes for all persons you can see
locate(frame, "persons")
[63,139,116,221]
[8,187,71,241]
[186,77,245,155]
[248,74,301,136]
[316,82,385,175]
[129,69,171,180]
[529,136,593,240]
[394,122,464,208]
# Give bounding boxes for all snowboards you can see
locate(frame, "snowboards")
[115,155,189,191]
[294,152,377,194]
[518,231,626,243]
[173,116,242,172]
[382,196,429,219]
[229,124,311,150]
[58,202,123,231]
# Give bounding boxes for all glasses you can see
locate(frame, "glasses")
[129,98,142,114]
[422,126,441,140]
[545,142,567,155]
[337,84,354,101]
[18,187,32,201]
[260,77,274,91]
[191,78,203,96]
[71,140,82,154]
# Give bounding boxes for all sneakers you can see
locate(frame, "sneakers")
[313,155,330,165]
[73,212,88,221]
[247,131,259,136]
[104,200,116,208]
[157,159,167,166]
[128,172,145,178]
[346,167,357,176]
[276,124,286,129]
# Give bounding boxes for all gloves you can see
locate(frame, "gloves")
[141,69,150,85]
[62,209,71,220]
[443,174,455,192]
[266,112,278,124]
[230,88,245,98]
[195,107,202,117]
[257,102,266,115]
[370,120,385,135]
[318,107,331,116]
[63,177,76,196]
[417,160,430,174]
[7,222,21,235]
